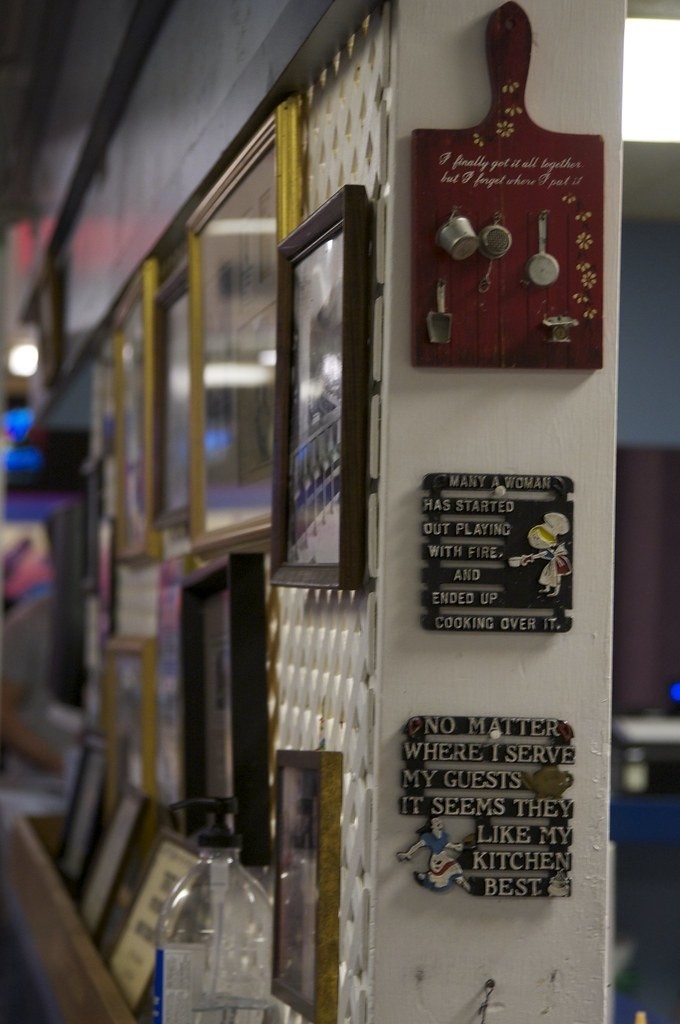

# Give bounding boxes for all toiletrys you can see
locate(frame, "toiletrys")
[159,795,274,1023]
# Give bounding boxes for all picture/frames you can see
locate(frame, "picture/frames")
[45,97,304,1010]
[268,748,343,1024]
[268,181,373,588]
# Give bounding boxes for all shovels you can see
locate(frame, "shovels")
[427,277,454,344]
